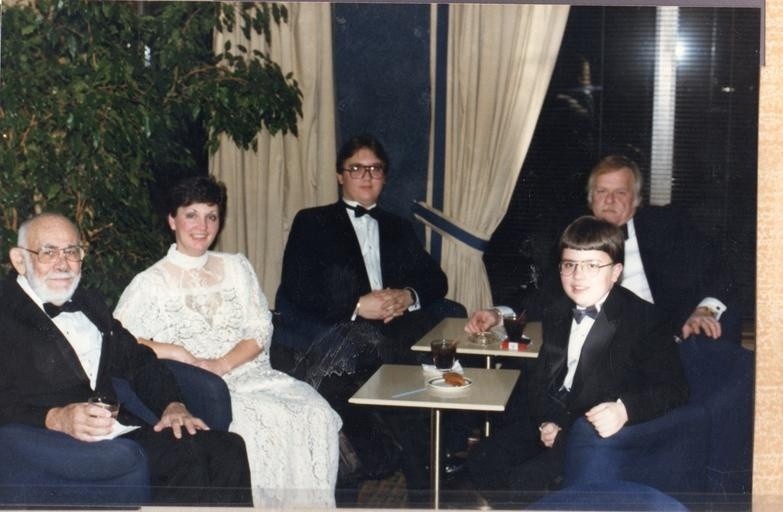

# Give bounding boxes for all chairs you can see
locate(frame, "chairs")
[713,279,742,341]
[283,295,466,445]
[270,312,395,506]
[562,333,755,490]
[524,479,685,512]
[0,358,233,507]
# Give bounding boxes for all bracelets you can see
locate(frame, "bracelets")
[492,309,502,324]
[221,358,232,376]
[407,288,416,306]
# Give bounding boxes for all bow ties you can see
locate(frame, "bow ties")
[44,300,82,318]
[572,304,596,323]
[354,205,381,221]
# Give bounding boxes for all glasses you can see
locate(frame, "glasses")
[344,164,387,180]
[17,245,84,264]
[559,259,617,277]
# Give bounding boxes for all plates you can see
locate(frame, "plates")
[426,378,474,390]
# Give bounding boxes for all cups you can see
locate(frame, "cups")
[88,395,121,420]
[504,316,526,341]
[430,339,457,369]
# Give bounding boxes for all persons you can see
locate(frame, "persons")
[0,213,251,507]
[112,174,343,507]
[270,133,463,477]
[467,214,688,509]
[465,154,738,340]
